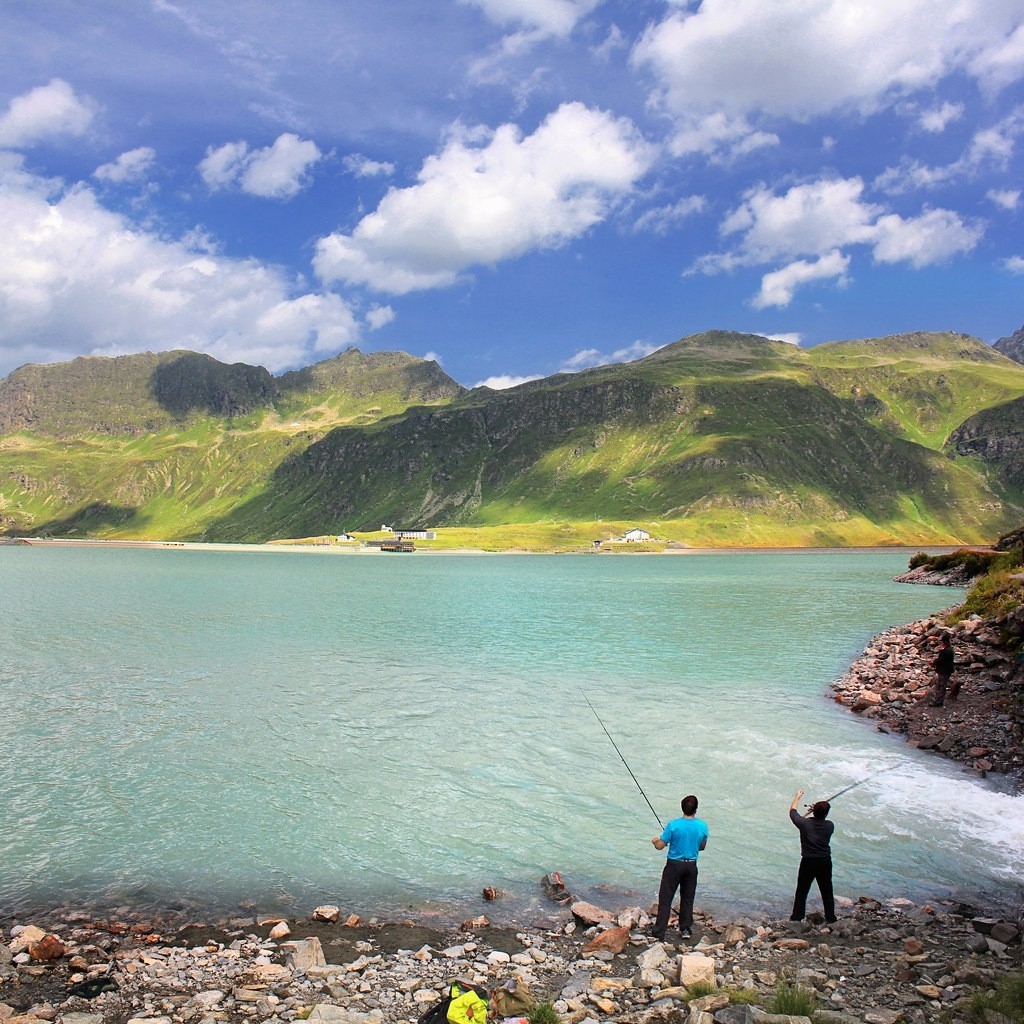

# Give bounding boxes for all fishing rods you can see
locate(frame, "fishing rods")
[577,685,664,829]
[803,753,917,817]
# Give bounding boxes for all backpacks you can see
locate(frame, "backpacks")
[417,980,489,1024]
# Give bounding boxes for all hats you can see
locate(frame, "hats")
[939,631,951,644]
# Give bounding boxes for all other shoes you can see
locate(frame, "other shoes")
[646,924,654,936]
[929,701,944,708]
[679,927,691,939]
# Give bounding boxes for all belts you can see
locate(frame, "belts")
[679,859,695,863]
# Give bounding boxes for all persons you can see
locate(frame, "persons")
[789,790,837,923]
[929,638,954,707]
[645,796,708,939]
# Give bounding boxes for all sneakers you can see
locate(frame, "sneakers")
[787,915,795,921]
[828,914,843,924]
